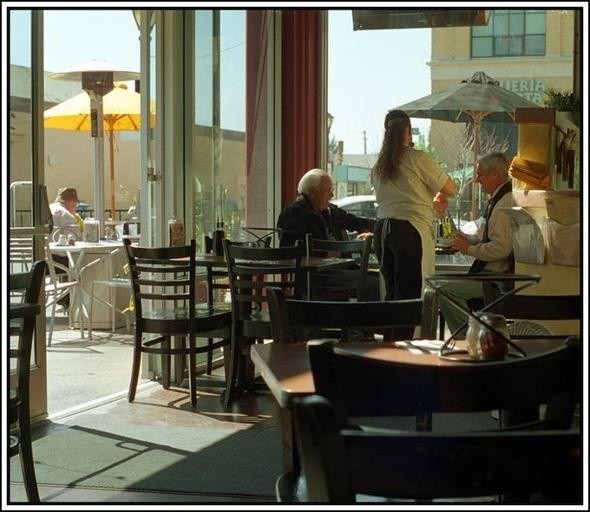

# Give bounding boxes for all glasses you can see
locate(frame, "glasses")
[328,185,336,194]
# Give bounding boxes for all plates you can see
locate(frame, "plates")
[434,243,452,249]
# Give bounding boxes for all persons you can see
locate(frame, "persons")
[435,153,520,340]
[49,187,83,308]
[278,169,376,300]
[371,110,459,342]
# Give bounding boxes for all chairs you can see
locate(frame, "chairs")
[8,225,143,346]
[119,237,232,407]
[223,236,304,410]
[9,258,41,503]
[296,394,583,504]
[306,234,374,307]
[195,233,273,377]
[264,282,437,347]
[275,338,582,505]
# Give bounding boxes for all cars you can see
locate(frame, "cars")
[331,195,378,220]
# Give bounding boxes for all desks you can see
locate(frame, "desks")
[171,253,356,401]
[249,333,583,475]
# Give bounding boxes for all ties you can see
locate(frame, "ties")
[482,201,491,226]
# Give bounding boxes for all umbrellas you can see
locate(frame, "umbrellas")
[43,79,156,240]
[388,73,540,226]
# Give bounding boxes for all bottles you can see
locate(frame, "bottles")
[466,311,510,360]
[58,235,75,246]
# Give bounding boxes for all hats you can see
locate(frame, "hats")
[54,187,81,204]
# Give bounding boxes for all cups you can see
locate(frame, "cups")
[213,230,224,257]
[439,239,449,245]
